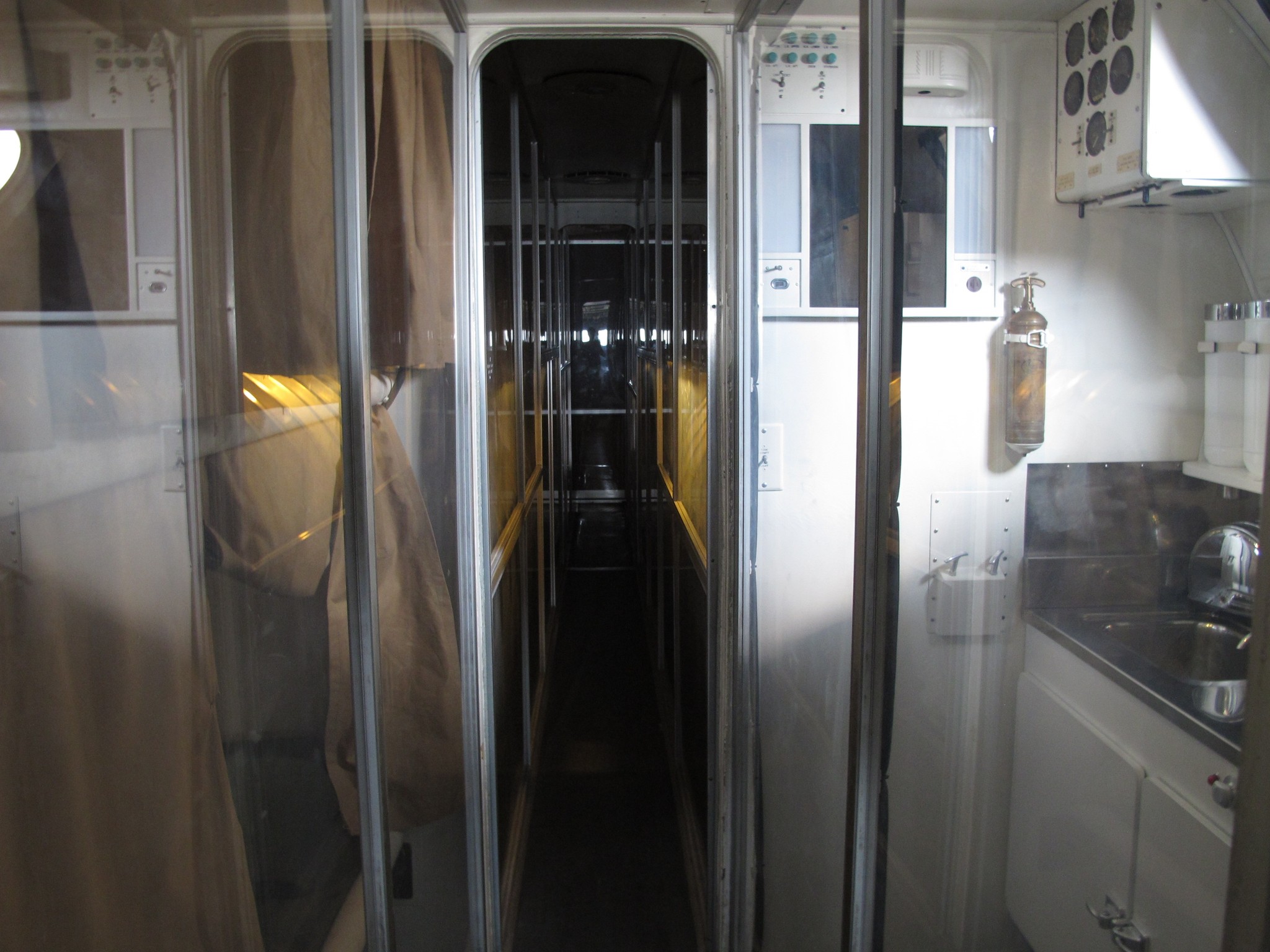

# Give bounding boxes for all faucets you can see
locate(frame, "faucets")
[1235,631,1252,650]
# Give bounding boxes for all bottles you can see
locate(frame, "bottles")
[1205,301,1269,482]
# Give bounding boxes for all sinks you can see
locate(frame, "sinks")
[1099,615,1250,683]
[1185,683,1253,726]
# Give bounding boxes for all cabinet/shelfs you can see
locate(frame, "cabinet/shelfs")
[1002,626,1239,951]
